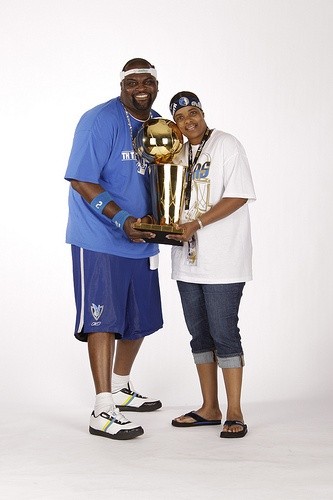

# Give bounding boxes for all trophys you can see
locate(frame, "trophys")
[130,117,183,247]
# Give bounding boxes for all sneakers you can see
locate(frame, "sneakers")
[112,388,162,412]
[89,404,144,440]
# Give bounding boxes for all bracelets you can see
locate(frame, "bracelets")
[110,208,133,233]
[194,217,204,230]
[89,190,113,214]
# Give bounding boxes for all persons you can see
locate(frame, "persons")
[164,91,249,438]
[64,58,167,440]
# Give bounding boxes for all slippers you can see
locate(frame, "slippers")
[220,420,247,438]
[172,411,221,427]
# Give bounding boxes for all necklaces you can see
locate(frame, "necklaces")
[120,97,153,156]
